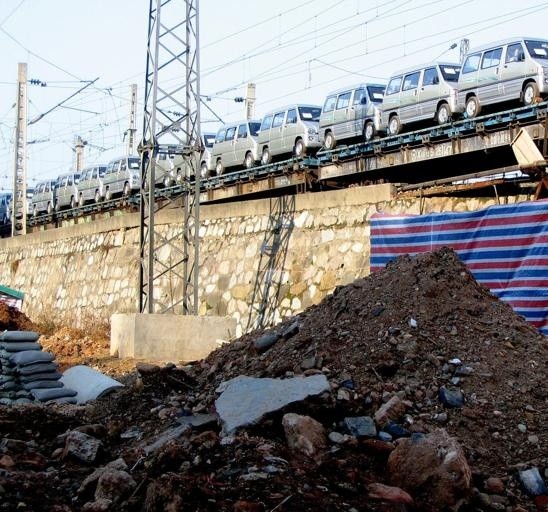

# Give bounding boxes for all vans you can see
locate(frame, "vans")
[316,82,389,151]
[457,36,546,119]
[1,131,217,223]
[210,118,262,176]
[380,62,478,136]
[255,103,321,166]
